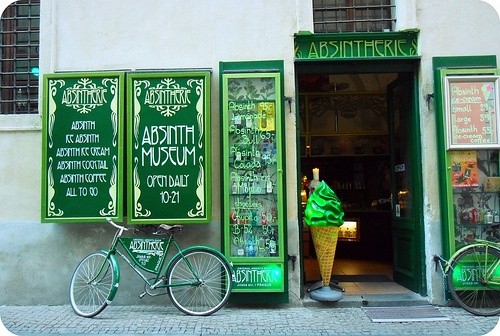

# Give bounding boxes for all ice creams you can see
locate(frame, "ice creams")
[304,180,345,286]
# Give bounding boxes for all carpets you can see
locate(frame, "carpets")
[361,305,450,323]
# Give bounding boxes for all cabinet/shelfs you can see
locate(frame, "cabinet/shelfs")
[448,149,499,256]
[223,72,284,263]
[299,91,392,158]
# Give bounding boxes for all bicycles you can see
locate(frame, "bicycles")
[442,237,500,317]
[68,216,235,318]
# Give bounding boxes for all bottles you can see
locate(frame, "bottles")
[459,209,500,224]
[233,107,277,257]
